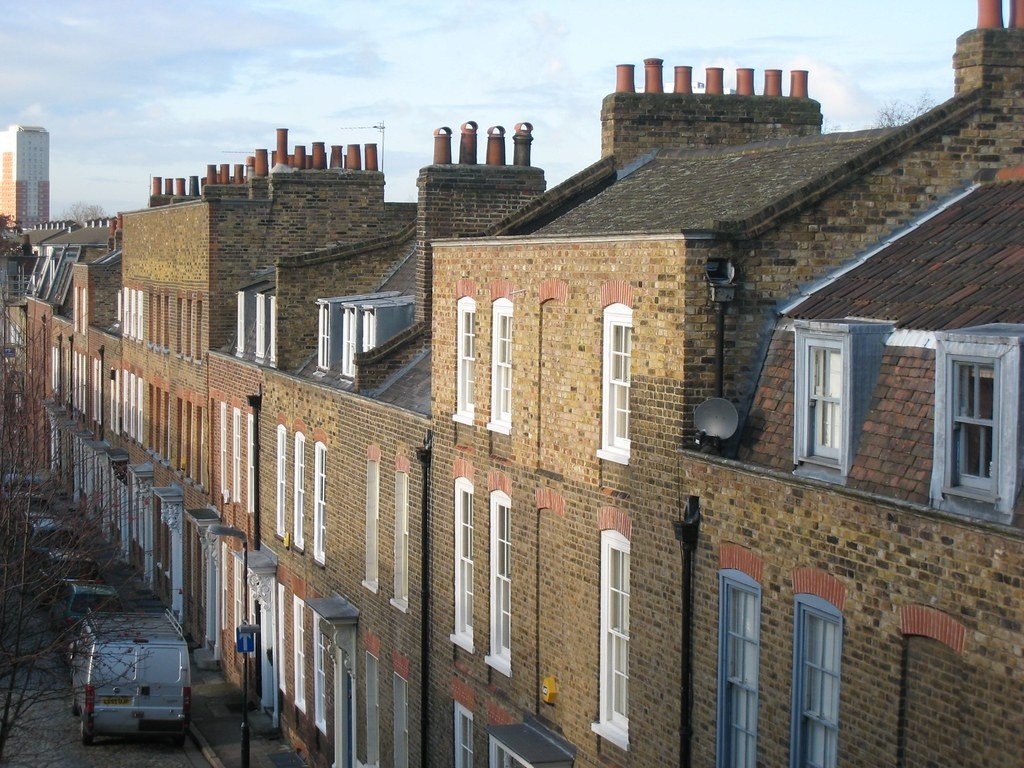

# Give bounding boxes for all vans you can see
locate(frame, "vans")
[68,608,191,749]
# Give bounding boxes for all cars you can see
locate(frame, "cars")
[2,473,123,636]
